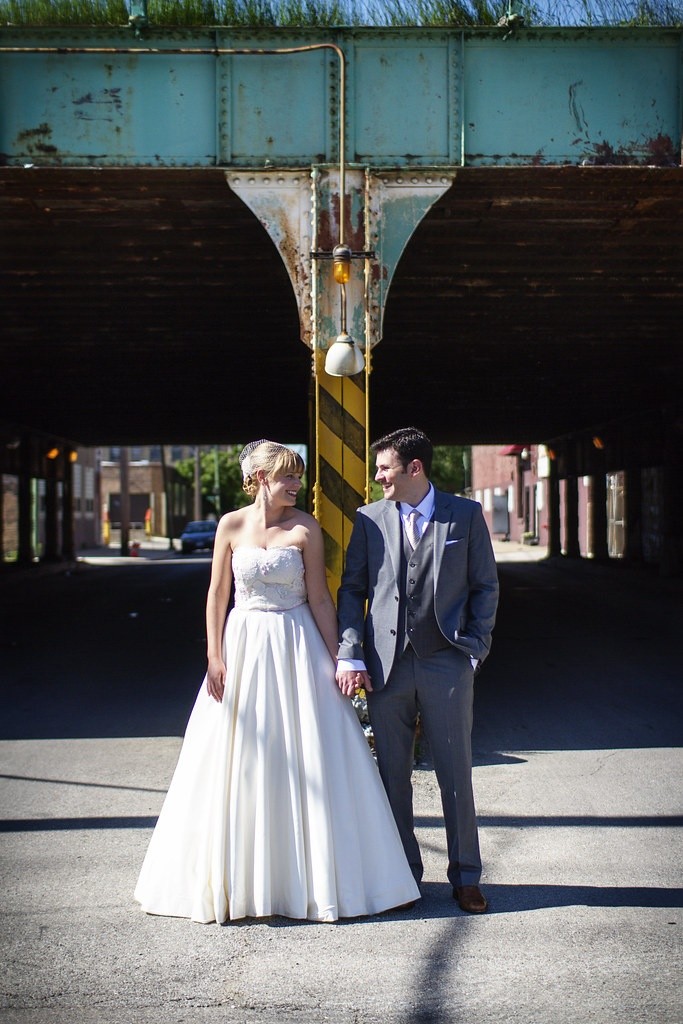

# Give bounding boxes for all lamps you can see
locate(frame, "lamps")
[307,245,378,376]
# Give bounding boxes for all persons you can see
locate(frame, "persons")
[134,440,422,923]
[335,428,499,912]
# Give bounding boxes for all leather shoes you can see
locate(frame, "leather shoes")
[452,885,488,913]
[396,882,420,911]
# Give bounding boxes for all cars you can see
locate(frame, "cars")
[179,520,217,554]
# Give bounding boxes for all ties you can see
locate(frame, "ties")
[407,512,421,551]
